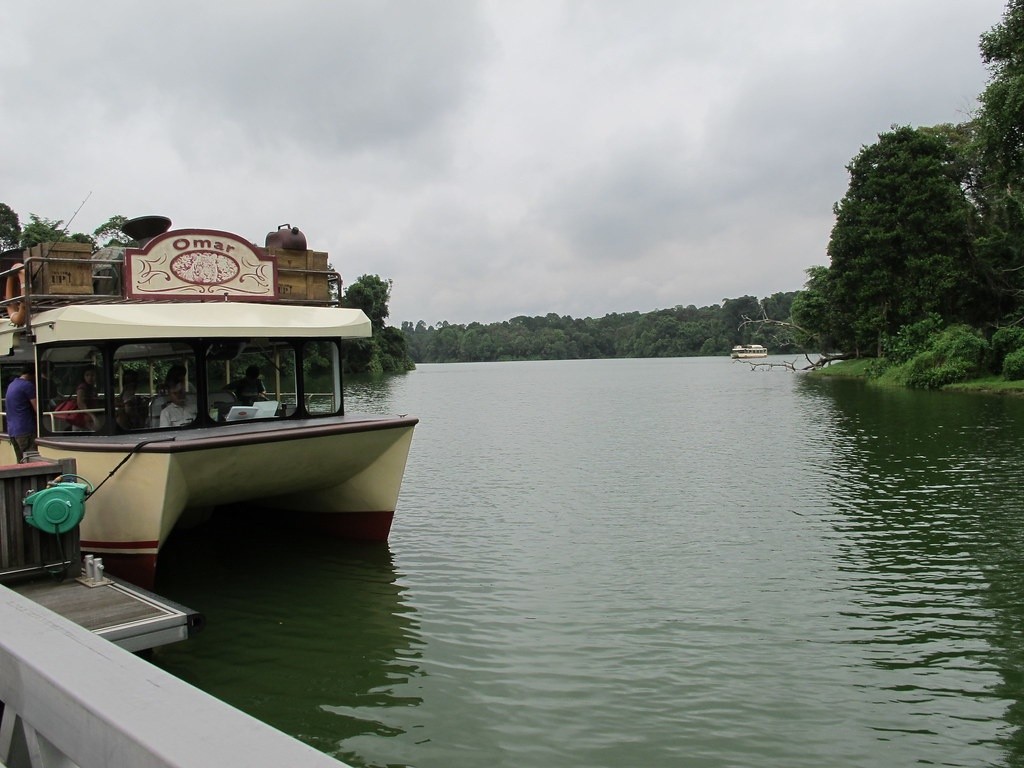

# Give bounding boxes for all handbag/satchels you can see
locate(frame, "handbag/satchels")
[53,387,94,427]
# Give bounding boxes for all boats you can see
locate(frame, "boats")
[730,344,768,359]
[0,226,419,601]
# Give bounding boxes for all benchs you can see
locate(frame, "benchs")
[148,391,236,429]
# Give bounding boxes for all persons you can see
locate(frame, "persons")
[5,363,36,464]
[46,365,100,427]
[157,363,197,427]
[120,369,141,413]
[222,365,266,393]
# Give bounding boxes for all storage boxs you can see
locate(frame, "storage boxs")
[254,245,329,300]
[24,242,94,294]
